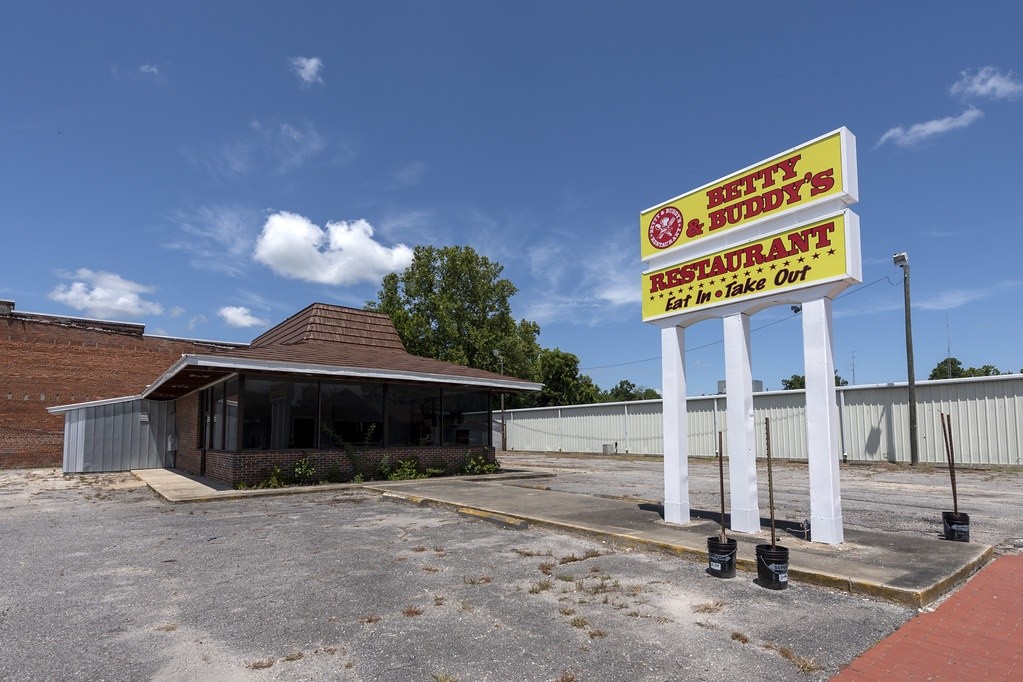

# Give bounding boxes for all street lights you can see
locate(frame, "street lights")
[892,252,918,465]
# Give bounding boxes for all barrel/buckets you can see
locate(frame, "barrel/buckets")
[706,537,737,579]
[941,511,969,542]
[755,544,789,589]
